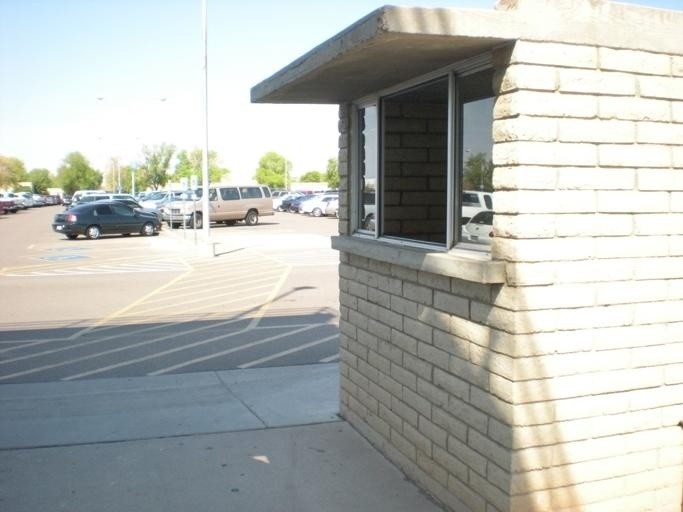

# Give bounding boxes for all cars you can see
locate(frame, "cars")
[460,189,494,245]
